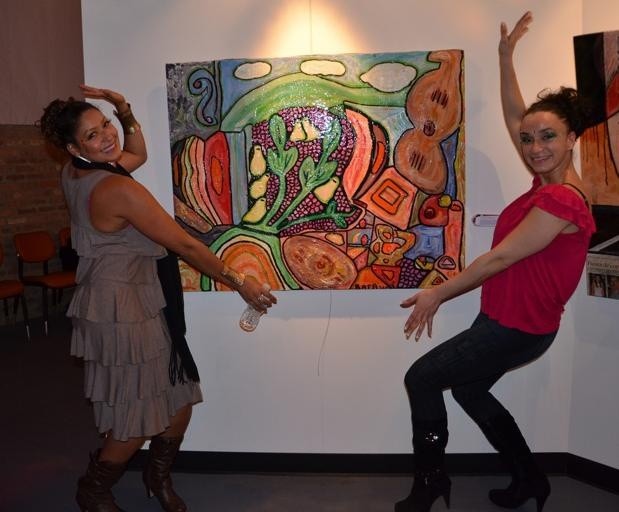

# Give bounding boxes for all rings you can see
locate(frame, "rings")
[257,294,268,304]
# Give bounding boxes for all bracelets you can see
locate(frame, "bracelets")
[210,260,247,295]
[112,102,141,136]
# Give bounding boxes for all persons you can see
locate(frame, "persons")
[38,82,280,511]
[379,10,598,512]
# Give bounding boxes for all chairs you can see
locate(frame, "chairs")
[11,230,78,337]
[58,227,79,317]
[0,234,33,344]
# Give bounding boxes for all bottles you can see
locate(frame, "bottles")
[239,283,271,331]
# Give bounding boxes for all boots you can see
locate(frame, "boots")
[76,449,129,512]
[393,416,452,512]
[143,437,187,512]
[480,413,551,512]
